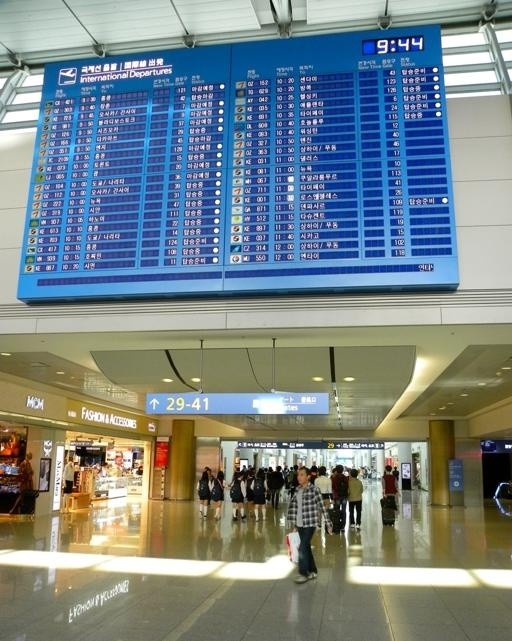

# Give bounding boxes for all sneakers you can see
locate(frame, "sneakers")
[356,523,360,528]
[308,571,317,579]
[350,523,355,527]
[293,575,308,583]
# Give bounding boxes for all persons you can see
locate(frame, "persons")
[18,452,35,516]
[205,467,214,506]
[61,457,75,515]
[212,471,226,521]
[85,460,144,480]
[313,466,332,529]
[246,469,256,519]
[197,471,209,521]
[381,465,399,515]
[230,462,377,509]
[391,466,399,487]
[331,465,348,535]
[250,470,267,522]
[347,469,363,531]
[39,459,50,491]
[229,472,247,521]
[286,468,334,584]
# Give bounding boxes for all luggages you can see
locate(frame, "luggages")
[382,508,395,525]
[326,508,342,534]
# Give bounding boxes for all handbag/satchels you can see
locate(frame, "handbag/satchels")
[380,495,397,510]
[285,531,301,562]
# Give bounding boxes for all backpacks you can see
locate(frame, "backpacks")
[211,479,223,501]
[230,483,241,499]
[199,480,209,496]
[253,479,264,495]
